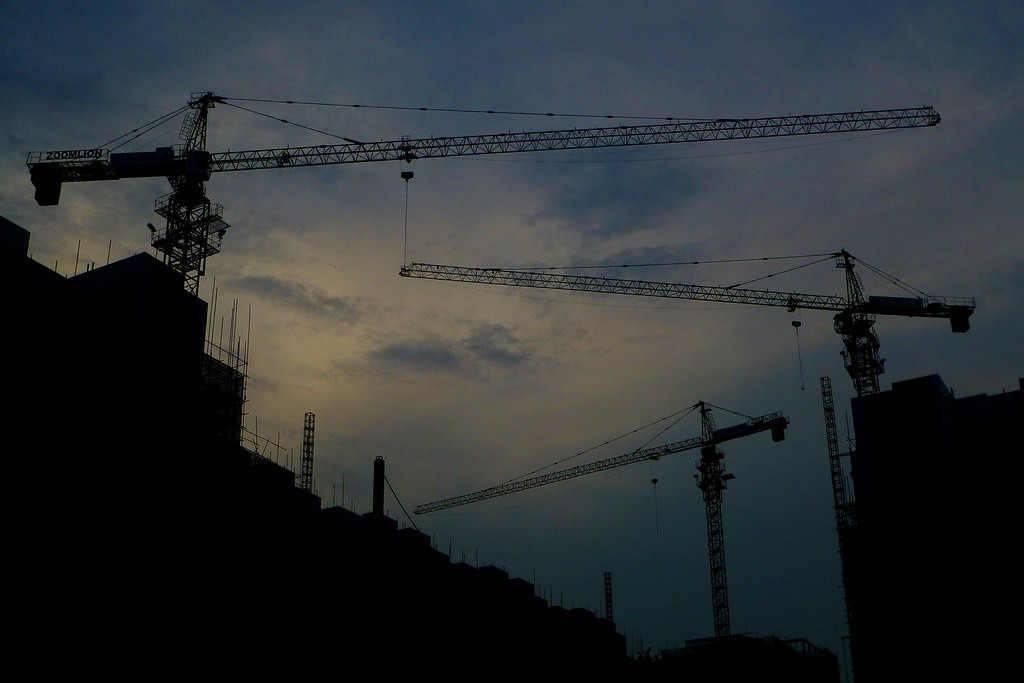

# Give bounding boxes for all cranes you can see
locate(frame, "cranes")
[395,242,982,369]
[24,82,943,287]
[407,395,792,642]
